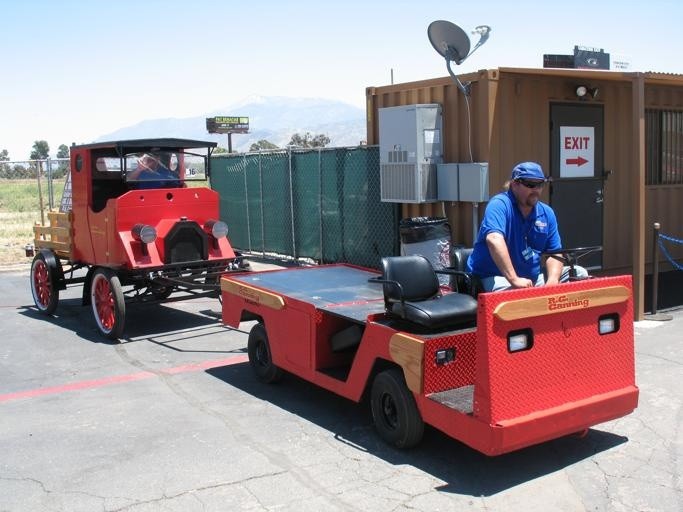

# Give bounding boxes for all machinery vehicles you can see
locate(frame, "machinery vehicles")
[219,245,641,450]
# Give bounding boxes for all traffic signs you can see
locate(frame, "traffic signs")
[215,115,249,129]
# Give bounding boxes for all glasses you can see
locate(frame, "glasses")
[515,180,545,188]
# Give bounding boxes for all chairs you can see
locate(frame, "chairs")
[367,254,478,330]
[444,247,474,293]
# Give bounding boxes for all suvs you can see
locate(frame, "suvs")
[21,139,250,337]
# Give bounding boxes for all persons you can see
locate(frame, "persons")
[126,151,180,189]
[464,162,590,292]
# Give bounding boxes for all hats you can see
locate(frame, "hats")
[511,161,546,180]
